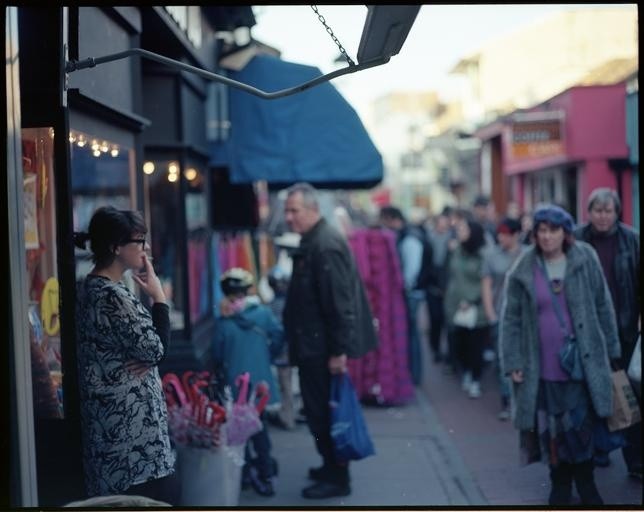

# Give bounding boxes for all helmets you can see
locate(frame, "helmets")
[220,267,254,294]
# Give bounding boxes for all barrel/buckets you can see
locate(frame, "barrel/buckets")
[178,444,244,507]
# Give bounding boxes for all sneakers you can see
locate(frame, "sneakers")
[462,370,481,399]
[301,465,351,499]
[549,474,573,504]
[575,476,611,506]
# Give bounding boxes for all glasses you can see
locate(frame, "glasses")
[124,236,148,250]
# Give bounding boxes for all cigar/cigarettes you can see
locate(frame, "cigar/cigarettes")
[148,256,154,260]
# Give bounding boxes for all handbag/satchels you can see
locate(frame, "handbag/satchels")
[601,369,640,433]
[558,333,583,383]
[328,372,377,461]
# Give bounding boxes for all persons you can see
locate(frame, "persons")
[66,206,178,507]
[379,188,641,508]
[209,183,379,498]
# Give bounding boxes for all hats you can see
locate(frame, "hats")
[532,204,574,233]
